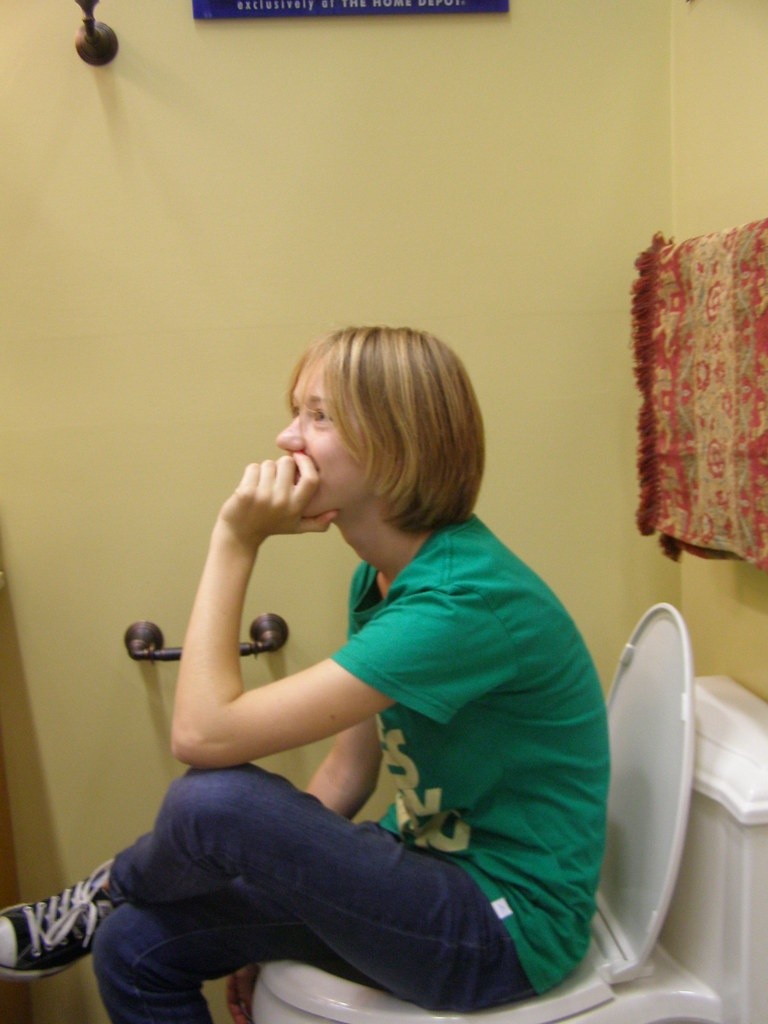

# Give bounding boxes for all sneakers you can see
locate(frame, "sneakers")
[0,859,116,980]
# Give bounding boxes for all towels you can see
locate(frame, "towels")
[629,220,768,571]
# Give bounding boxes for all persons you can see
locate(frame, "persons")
[0,323,613,1024]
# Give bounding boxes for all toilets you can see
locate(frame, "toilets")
[249,600,767,1024]
